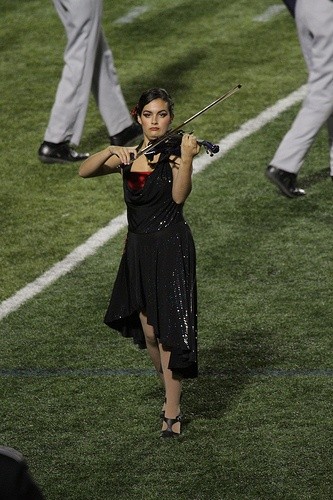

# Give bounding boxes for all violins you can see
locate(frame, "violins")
[143,129,220,164]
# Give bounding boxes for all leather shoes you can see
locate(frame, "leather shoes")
[110,124,144,146]
[38,141,89,165]
[265,165,306,198]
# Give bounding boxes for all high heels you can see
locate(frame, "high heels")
[161,413,182,438]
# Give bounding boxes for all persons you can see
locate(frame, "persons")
[80,87,201,441]
[0,442,46,500]
[266,0,333,201]
[38,0,148,165]
